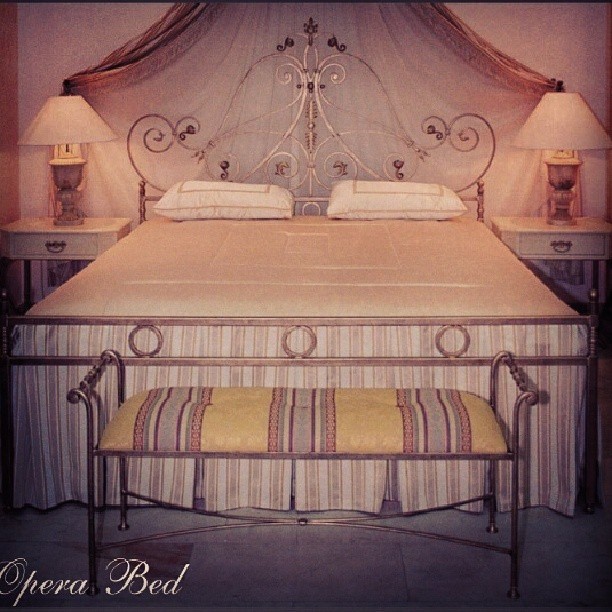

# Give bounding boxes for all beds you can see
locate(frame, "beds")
[66,349,538,602]
[4,19,601,522]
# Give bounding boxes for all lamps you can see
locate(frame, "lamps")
[18,96,119,225]
[511,92,611,225]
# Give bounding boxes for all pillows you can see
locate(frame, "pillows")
[153,179,295,221]
[326,180,468,221]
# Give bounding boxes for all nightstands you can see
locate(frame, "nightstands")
[489,214,611,301]
[3,216,132,315]
